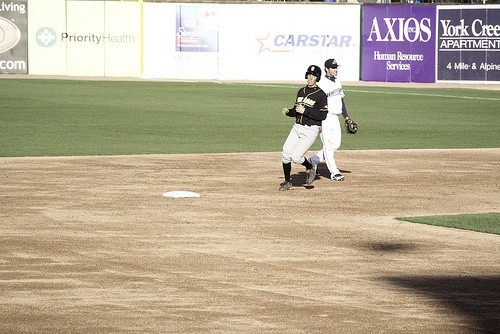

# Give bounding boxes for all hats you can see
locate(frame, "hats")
[324,58,341,68]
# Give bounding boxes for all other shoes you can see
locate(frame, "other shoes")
[279,181,292,191]
[308,158,318,175]
[306,164,317,184]
[331,174,345,181]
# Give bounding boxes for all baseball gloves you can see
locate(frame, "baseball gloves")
[345,119,359,134]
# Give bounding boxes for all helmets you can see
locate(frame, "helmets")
[305,65,322,82]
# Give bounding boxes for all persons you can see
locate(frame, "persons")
[307,58,358,182]
[278,64,327,188]
